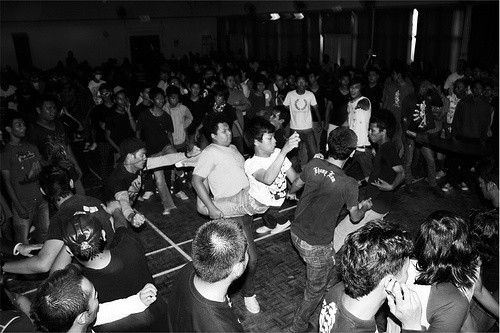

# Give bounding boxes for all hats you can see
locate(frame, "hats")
[116,137,145,163]
[62,209,103,251]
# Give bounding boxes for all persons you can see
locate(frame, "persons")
[166,219,249,333]
[0,50,499,333]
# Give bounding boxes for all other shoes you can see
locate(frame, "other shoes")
[88,142,97,150]
[436,170,447,179]
[441,183,453,192]
[256,226,273,233]
[460,180,469,191]
[303,322,319,333]
[175,191,189,200]
[244,294,260,314]
[82,142,90,152]
[271,220,291,235]
[142,191,154,199]
